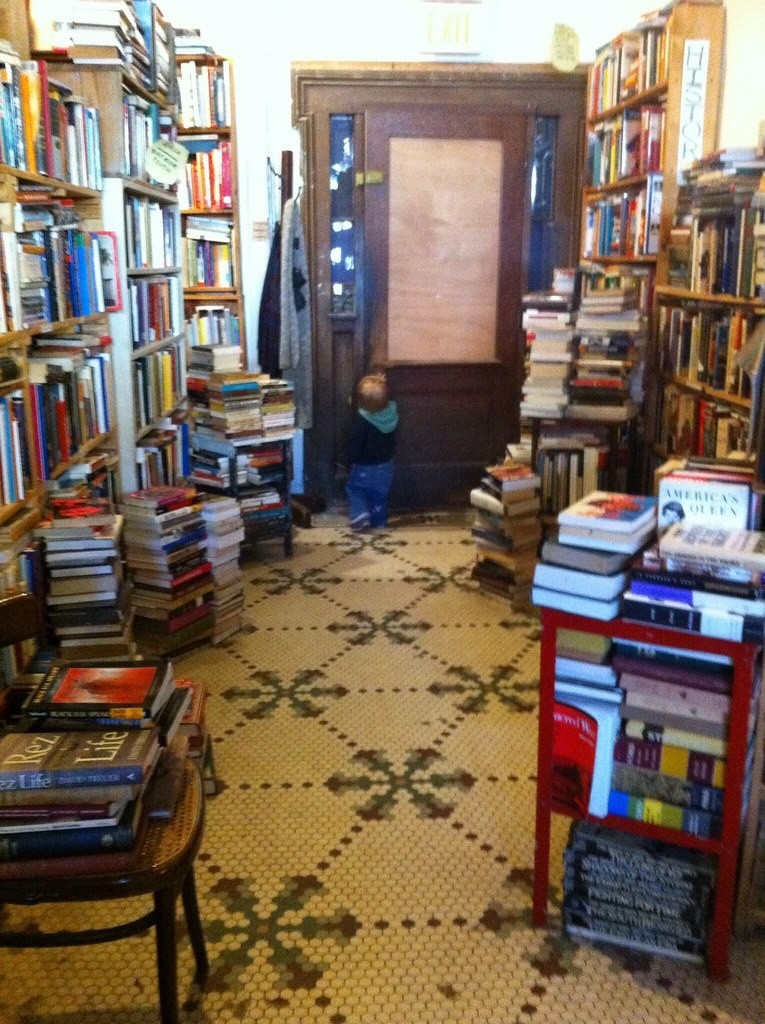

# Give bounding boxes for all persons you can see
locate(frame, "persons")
[345,369,400,531]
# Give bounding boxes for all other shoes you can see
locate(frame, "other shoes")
[349,511,372,531]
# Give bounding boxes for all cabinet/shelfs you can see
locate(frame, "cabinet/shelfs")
[532,0,764,980]
[0,0,293,680]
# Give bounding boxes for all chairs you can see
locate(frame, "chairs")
[0,593,211,1024]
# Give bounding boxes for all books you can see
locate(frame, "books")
[467,260,657,608]
[0,508,140,692]
[525,451,765,962]
[0,659,207,879]
[575,26,668,258]
[648,123,765,461]
[115,486,245,663]
[0,0,245,506]
[182,340,297,545]
